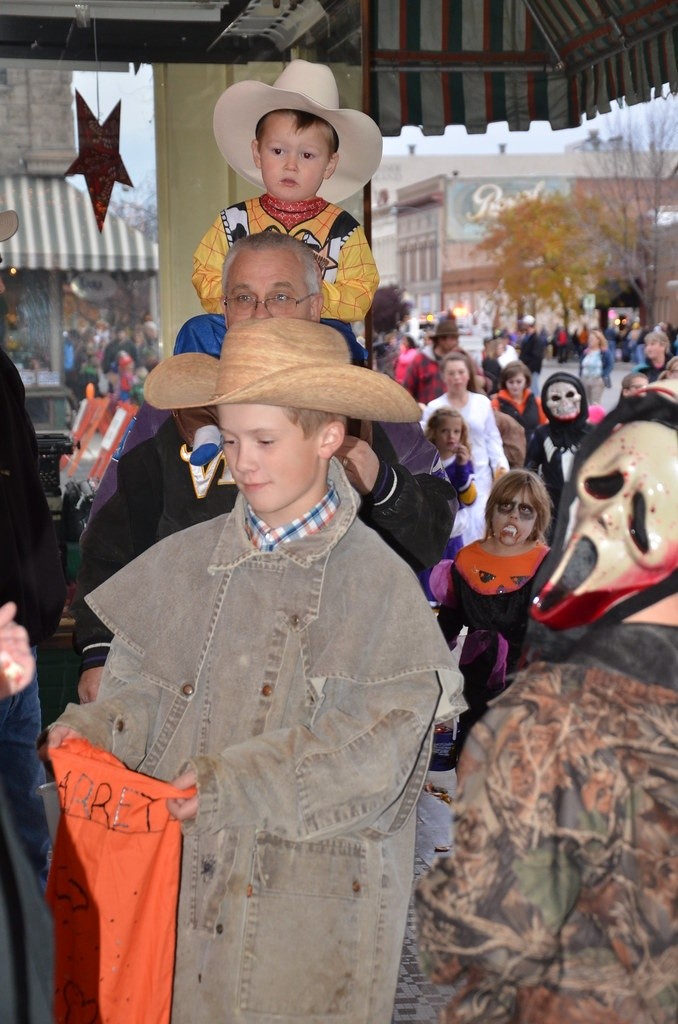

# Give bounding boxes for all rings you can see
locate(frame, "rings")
[342,458,349,468]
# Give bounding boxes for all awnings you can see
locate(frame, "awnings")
[1,178,160,274]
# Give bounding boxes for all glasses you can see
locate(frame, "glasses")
[224,292,319,316]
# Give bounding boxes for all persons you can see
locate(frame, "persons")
[188,60,387,322]
[72,232,460,887]
[37,314,469,1024]
[0,345,68,887]
[0,309,160,426]
[380,294,678,1024]
[0,600,58,1024]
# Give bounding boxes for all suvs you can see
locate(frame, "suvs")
[22,381,80,499]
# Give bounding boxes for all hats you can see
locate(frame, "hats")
[519,314,536,327]
[428,320,471,340]
[144,319,424,423]
[213,59,382,204]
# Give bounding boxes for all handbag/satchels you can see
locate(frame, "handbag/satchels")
[40,739,197,1024]
